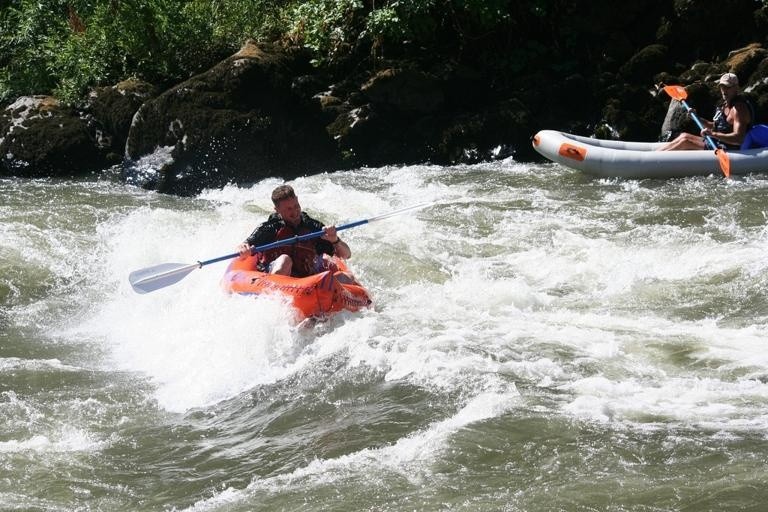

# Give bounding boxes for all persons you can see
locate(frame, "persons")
[660,73,753,152]
[236,184,351,278]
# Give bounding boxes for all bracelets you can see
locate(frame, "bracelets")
[332,237,341,244]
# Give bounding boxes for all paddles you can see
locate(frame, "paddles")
[663,85,731,177]
[130,201,436,295]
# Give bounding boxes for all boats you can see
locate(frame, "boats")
[528,128,768,178]
[225,246,372,319]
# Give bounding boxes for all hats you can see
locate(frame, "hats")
[717,73,737,88]
